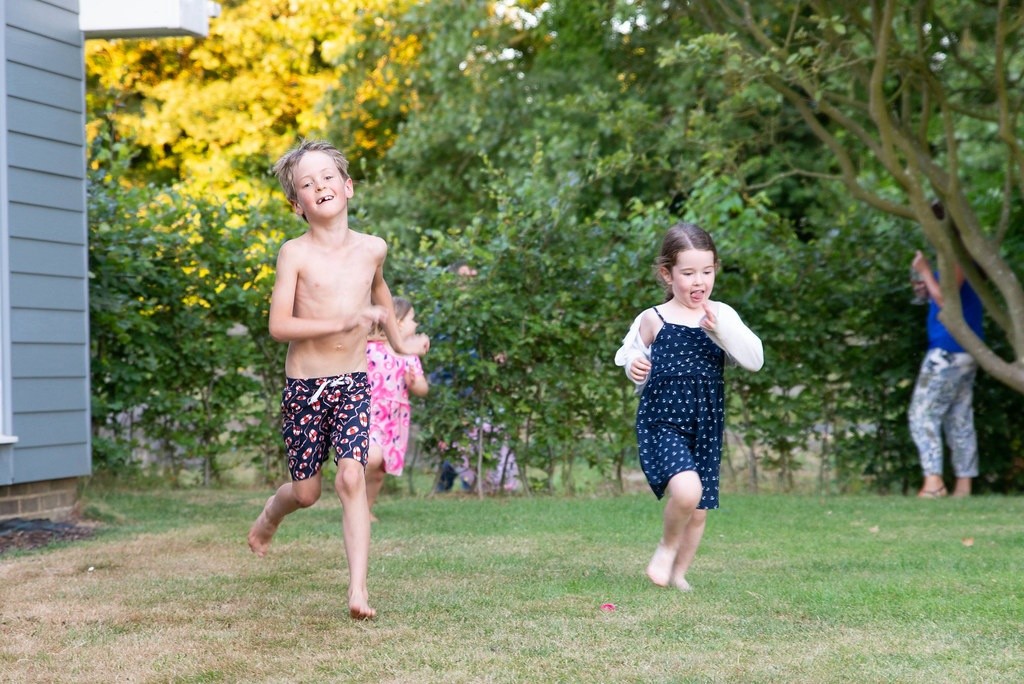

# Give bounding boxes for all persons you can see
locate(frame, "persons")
[911,205,982,501]
[364,261,508,525]
[614,225,766,592]
[247,141,428,617]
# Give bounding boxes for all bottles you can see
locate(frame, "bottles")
[909,266,926,303]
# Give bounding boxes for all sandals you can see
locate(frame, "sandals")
[915,488,950,500]
